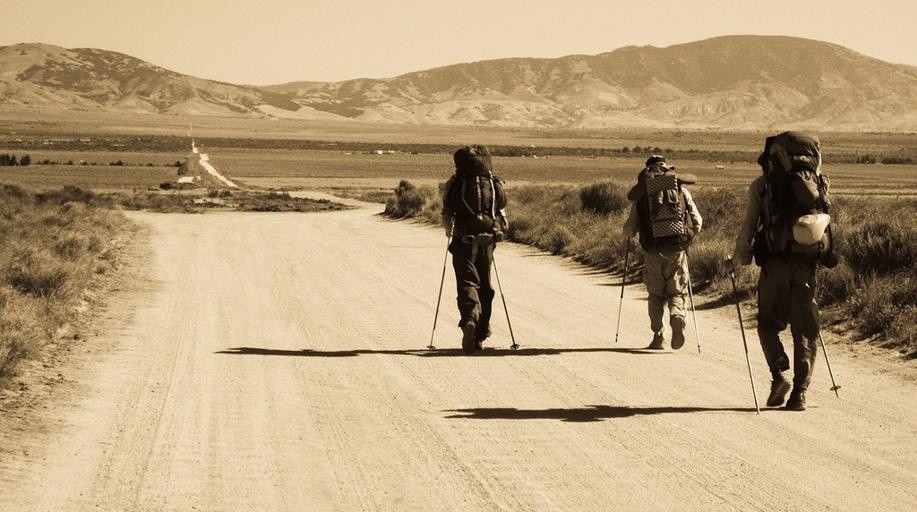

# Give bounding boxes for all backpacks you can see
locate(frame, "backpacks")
[453,144,504,246]
[754,130,832,268]
[627,163,696,254]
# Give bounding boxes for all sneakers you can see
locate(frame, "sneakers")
[457,316,486,353]
[648,331,665,349]
[766,377,792,406]
[670,314,685,349]
[786,399,805,411]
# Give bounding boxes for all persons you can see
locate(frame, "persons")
[737,136,831,410]
[442,159,507,354]
[624,155,703,349]
